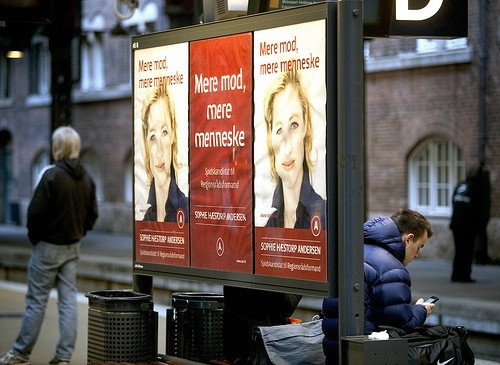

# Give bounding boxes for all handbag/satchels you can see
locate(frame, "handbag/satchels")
[403,325,475,365]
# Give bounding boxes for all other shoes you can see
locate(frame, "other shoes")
[0,352,30,365]
[451,275,475,284]
[49,357,71,365]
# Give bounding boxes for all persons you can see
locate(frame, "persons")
[265,67,326,230]
[449,169,490,283]
[322,208,459,365]
[0,126,99,365]
[143,78,188,222]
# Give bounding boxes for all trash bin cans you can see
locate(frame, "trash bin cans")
[172,292,223,362]
[342,335,409,365]
[84,290,153,364]
